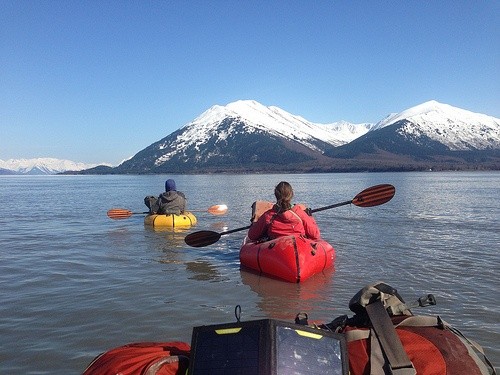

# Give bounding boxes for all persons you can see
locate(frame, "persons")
[144,179,185,216]
[248,182,319,241]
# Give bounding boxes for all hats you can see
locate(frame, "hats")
[165,179,175,191]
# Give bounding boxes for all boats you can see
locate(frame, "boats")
[143,206,196,229]
[238,201,337,284]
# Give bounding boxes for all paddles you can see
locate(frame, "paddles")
[184,183,396,248]
[106,204,228,221]
